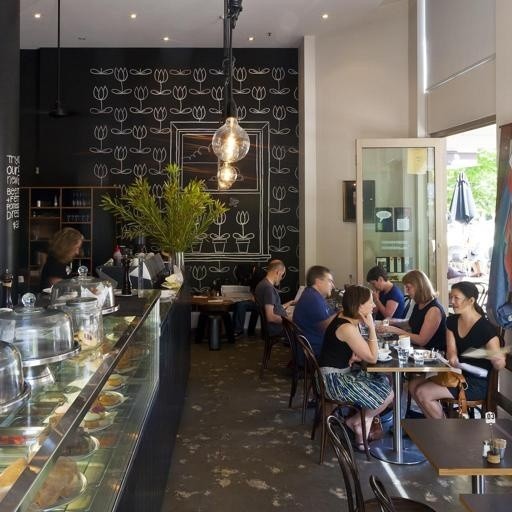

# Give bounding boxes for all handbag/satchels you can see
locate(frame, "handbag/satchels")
[431,369,469,414]
[330,404,383,439]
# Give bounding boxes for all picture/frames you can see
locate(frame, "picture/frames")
[341,180,376,224]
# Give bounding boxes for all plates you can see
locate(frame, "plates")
[19,325,157,512]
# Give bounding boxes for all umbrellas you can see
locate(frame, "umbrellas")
[448,166,481,228]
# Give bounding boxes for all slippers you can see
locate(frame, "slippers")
[351,442,370,452]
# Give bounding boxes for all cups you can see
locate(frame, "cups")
[493,446,505,459]
[36,200,41,208]
[361,318,442,366]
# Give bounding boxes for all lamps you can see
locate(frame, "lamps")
[210,1,251,190]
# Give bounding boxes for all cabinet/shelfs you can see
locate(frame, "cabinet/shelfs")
[19,186,123,292]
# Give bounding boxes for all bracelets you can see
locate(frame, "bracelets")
[367,338,379,342]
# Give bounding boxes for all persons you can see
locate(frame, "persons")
[291,265,342,370]
[376,269,448,353]
[252,257,297,348]
[315,285,394,452]
[366,265,406,324]
[39,228,86,298]
[406,280,507,418]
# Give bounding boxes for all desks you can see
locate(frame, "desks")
[362,347,452,466]
[399,418,512,511]
[191,295,250,352]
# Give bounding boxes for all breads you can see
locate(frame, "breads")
[0,373,123,512]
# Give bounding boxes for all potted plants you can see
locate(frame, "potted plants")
[97,161,232,272]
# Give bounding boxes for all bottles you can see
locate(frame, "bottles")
[216,290,219,296]
[331,288,343,311]
[482,440,490,458]
[121,254,133,296]
[113,244,122,265]
[53,195,58,208]
[16,275,27,306]
[1,268,15,309]
[32,211,36,218]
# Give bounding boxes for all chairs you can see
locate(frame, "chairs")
[253,294,374,465]
[324,414,439,512]
[442,334,505,418]
[401,294,411,319]
